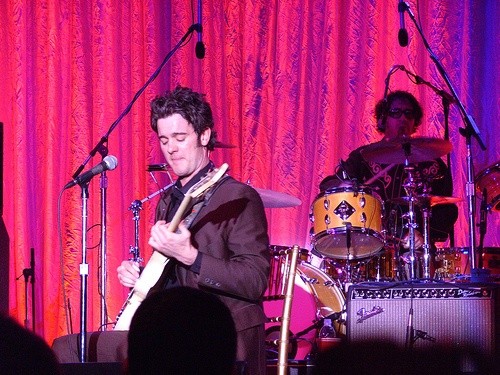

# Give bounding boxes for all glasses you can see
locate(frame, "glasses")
[386,107,418,119]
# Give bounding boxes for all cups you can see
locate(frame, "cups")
[315,338,340,352]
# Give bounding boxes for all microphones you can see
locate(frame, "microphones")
[349,150,361,187]
[195,0,202,59]
[398,0,408,47]
[64,155,118,188]
[405,308,413,348]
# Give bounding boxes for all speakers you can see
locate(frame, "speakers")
[345,284,500,357]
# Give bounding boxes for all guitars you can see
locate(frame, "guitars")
[113,162,229,330]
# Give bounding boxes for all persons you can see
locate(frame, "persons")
[117,87,272,375]
[124,285,238,375]
[337,90,459,253]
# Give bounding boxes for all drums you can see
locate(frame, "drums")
[436,246,500,283]
[325,242,396,300]
[308,186,387,260]
[473,160,500,212]
[264,257,347,359]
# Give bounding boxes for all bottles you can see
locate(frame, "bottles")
[320,319,335,338]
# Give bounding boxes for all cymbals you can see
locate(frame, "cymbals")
[383,192,462,207]
[361,137,453,165]
[252,186,302,208]
